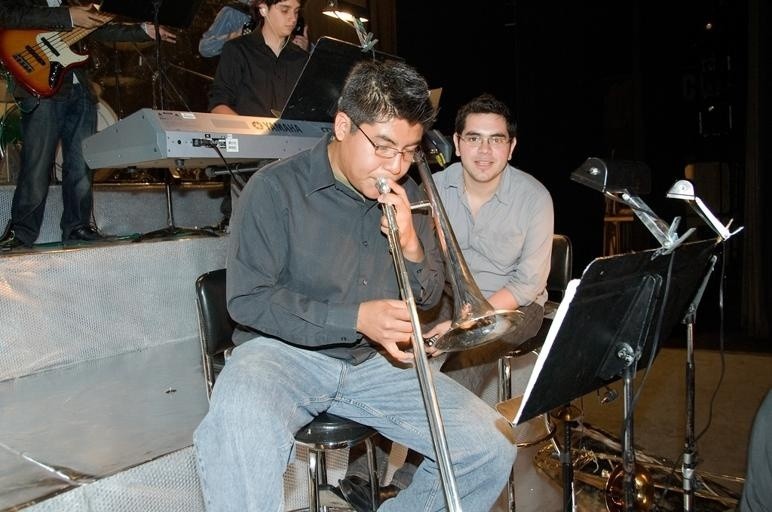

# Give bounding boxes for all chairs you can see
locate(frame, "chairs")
[193,268,379,512]
[495,233,574,512]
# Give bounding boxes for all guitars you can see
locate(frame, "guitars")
[0,12,117,98]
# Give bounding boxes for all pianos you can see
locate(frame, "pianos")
[83,108,452,169]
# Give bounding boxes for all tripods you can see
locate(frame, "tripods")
[130,12,221,244]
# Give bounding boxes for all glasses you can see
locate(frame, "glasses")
[457,133,514,147]
[344,111,423,164]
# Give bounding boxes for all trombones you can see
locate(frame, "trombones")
[369,145,527,512]
[536,442,746,512]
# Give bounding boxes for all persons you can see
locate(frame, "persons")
[190,56,518,512]
[206,0,315,234]
[1,0,183,250]
[198,0,316,57]
[737,387,772,512]
[336,91,556,511]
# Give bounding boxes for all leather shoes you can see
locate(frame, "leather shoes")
[333,474,403,512]
[61,226,114,249]
[0,230,33,252]
